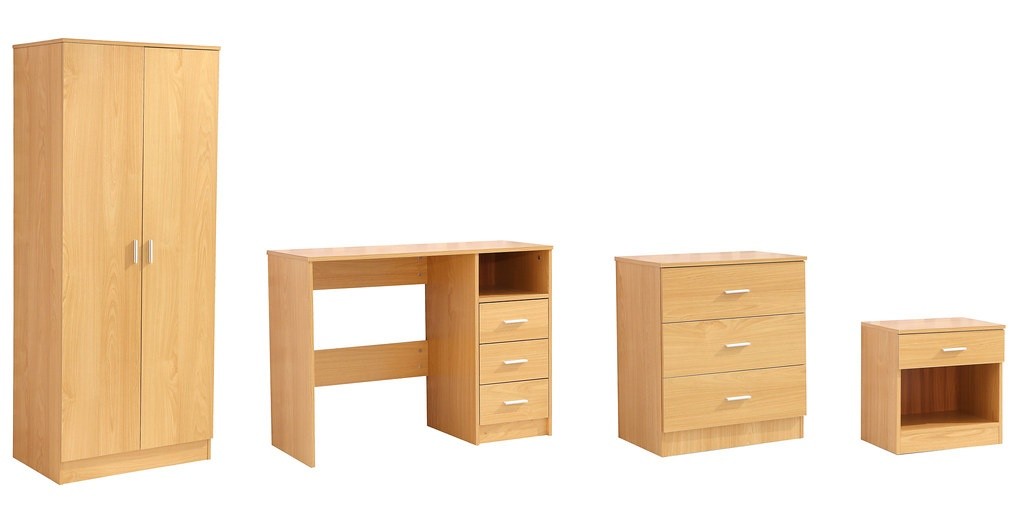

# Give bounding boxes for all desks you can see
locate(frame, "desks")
[267,240,555,468]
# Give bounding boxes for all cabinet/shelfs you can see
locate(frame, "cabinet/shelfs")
[614,248,806,458]
[862,317,1007,454]
[12,40,221,486]
